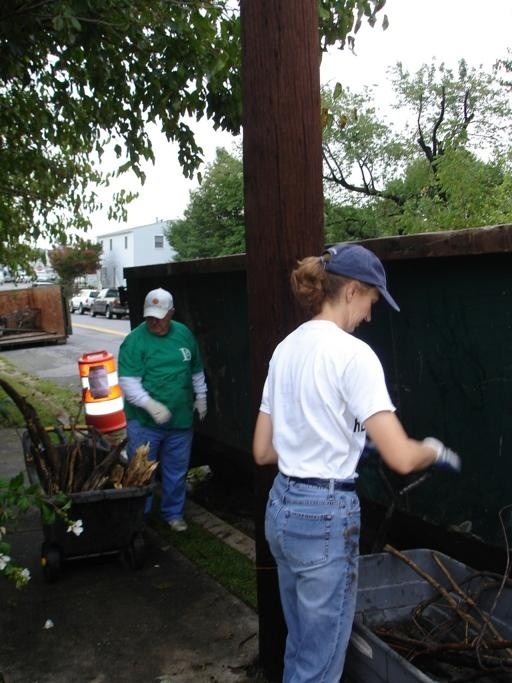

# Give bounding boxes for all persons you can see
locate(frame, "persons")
[250,238,464,681]
[117,286,209,532]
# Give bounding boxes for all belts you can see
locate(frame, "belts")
[281,472,355,492]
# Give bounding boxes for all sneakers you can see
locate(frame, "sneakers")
[168,518,188,531]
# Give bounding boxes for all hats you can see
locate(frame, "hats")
[143,288,175,319]
[321,242,402,311]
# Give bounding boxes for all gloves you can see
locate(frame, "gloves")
[144,399,172,425]
[192,399,208,421]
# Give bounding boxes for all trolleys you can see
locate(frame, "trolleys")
[24,418,154,566]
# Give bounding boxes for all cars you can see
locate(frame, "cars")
[0,263,58,285]
[68,287,143,319]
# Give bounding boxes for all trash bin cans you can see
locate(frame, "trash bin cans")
[78,351,127,433]
